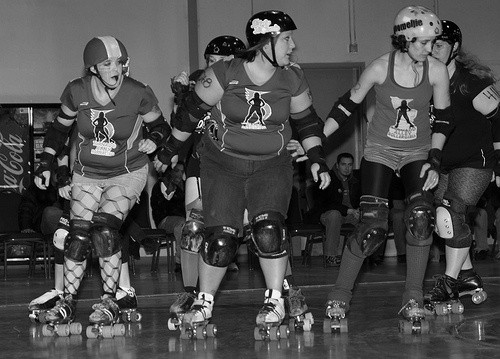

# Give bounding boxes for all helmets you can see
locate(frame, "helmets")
[394,5,442,41]
[84,35,128,68]
[245,9,296,47]
[434,20,462,53]
[205,35,245,58]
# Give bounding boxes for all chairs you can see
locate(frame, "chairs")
[0,185,497,281]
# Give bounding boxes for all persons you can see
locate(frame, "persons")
[286,7,453,321]
[28,39,168,325]
[427,16,500,303]
[151,10,332,324]
[318,152,364,263]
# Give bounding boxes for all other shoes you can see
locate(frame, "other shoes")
[227,262,238,270]
[325,255,341,266]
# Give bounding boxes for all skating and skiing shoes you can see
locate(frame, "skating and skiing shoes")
[456,272,488,304]
[423,273,464,316]
[254,288,290,341]
[178,296,217,340]
[323,287,351,335]
[286,289,314,332]
[39,291,82,337]
[28,289,64,320]
[86,295,125,338]
[168,288,198,331]
[115,287,142,322]
[393,292,429,335]
